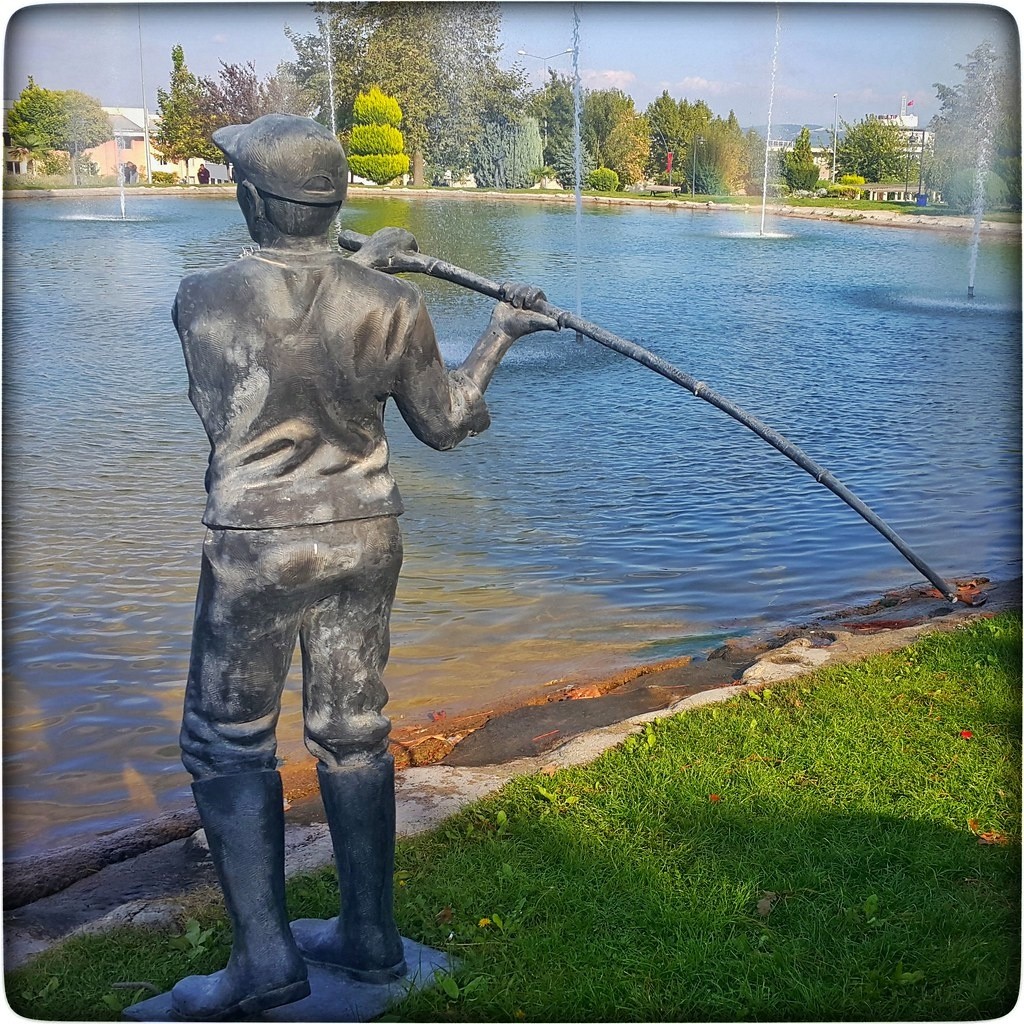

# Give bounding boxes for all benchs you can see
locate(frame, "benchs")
[646,185,681,197]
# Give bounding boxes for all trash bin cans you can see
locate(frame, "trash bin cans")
[912,193,929,207]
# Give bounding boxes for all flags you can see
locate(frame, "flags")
[907,100,913,106]
[666,152,673,173]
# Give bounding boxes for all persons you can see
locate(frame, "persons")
[198,164,210,184]
[168,115,559,1023]
[124,161,139,184]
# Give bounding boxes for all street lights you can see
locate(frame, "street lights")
[518,48,575,187]
[635,116,707,186]
